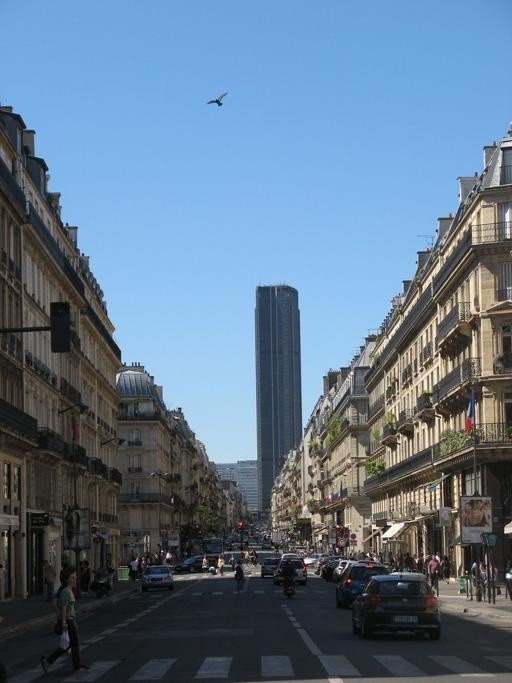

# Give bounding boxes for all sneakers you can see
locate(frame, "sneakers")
[40,656,52,673]
[73,665,92,673]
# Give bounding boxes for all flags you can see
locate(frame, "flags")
[337,484,343,500]
[466,389,475,433]
[327,493,334,505]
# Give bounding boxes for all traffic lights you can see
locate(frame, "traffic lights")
[240,520,243,527]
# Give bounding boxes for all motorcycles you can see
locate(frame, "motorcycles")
[92,567,118,599]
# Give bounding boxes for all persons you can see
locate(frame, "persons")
[79,559,90,595]
[41,559,56,599]
[467,500,490,526]
[471,563,486,584]
[41,566,90,673]
[464,500,475,528]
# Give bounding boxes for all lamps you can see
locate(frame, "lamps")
[101,436,125,447]
[57,401,89,416]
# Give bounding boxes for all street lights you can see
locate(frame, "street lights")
[150,472,169,529]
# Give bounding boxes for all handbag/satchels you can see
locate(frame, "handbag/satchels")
[54,621,73,636]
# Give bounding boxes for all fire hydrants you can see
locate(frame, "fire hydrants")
[474,586,483,602]
